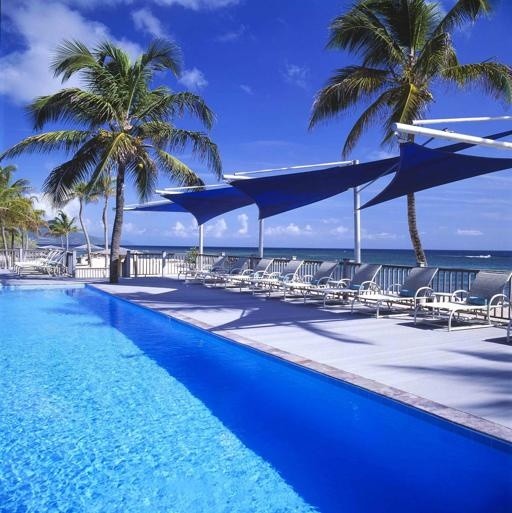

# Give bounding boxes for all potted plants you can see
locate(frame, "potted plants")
[184,245,198,269]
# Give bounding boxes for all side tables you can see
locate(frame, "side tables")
[433,292,456,316]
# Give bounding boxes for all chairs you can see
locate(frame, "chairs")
[350,267,439,319]
[14,249,69,278]
[182,255,275,293]
[247,260,382,310]
[413,270,512,331]
